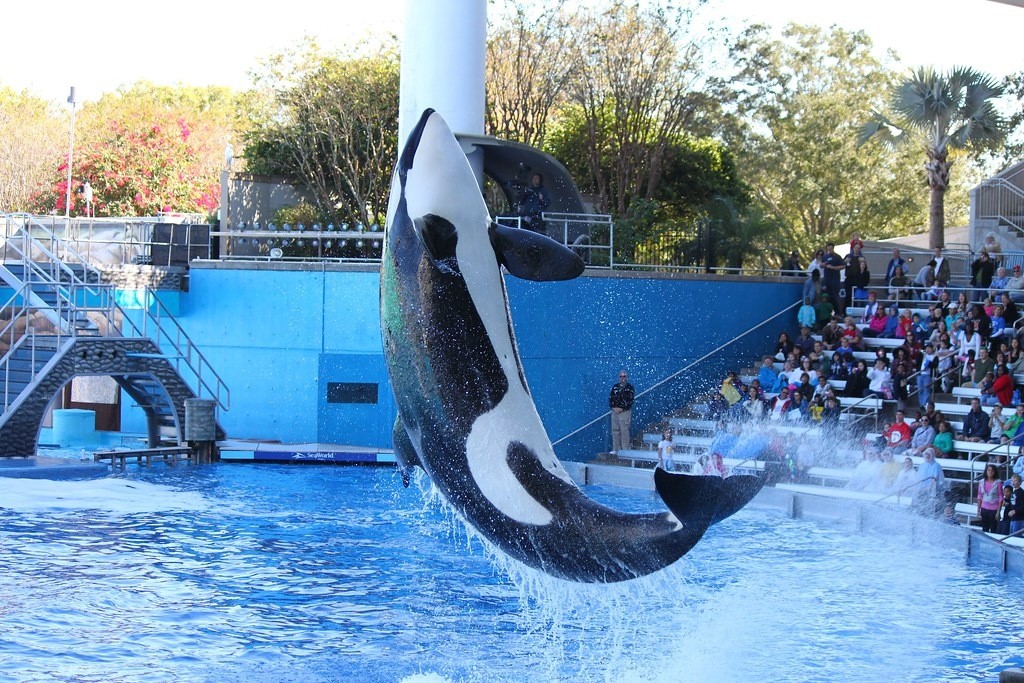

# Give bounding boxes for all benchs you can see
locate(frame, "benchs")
[835,448,1005,481]
[925,402,1018,418]
[668,419,823,441]
[763,393,883,419]
[772,363,887,372]
[822,350,893,364]
[641,433,713,456]
[807,466,976,505]
[812,335,929,350]
[691,403,857,431]
[896,417,972,433]
[845,307,1024,322]
[952,387,983,404]
[617,450,781,474]
[776,482,1004,531]
[741,375,846,390]
[865,432,1020,467]
[827,323,1018,338]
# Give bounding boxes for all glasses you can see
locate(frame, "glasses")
[782,391,788,395]
[929,308,935,312]
[921,419,928,422]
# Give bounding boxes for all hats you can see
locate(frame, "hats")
[712,389,722,396]
[986,232,996,241]
[1012,265,1020,271]
[946,302,958,309]
[993,402,1003,408]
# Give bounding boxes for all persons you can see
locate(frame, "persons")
[609,372,634,455]
[997,447,1023,537]
[930,246,951,285]
[845,444,917,497]
[914,259,937,300]
[975,463,1002,533]
[657,426,677,470]
[708,320,983,427]
[708,419,817,479]
[973,339,1024,408]
[968,251,994,301]
[797,230,1024,338]
[821,243,846,288]
[887,266,912,305]
[956,398,1024,447]
[872,401,952,459]
[910,447,947,517]
[515,173,551,236]
[691,452,726,476]
